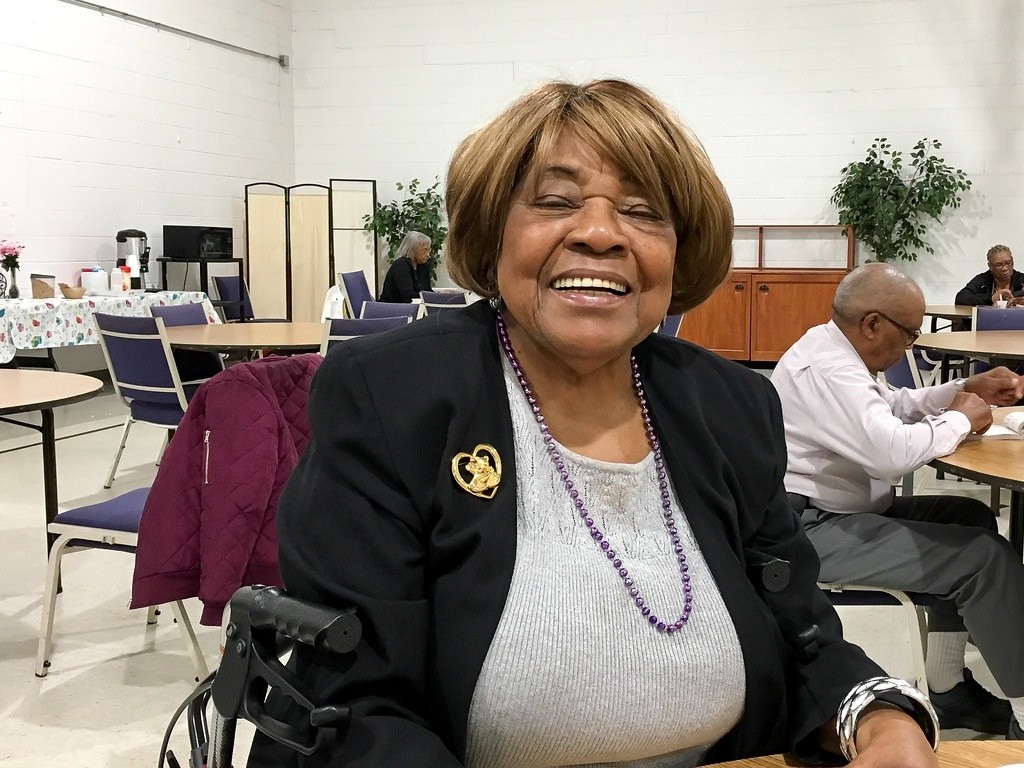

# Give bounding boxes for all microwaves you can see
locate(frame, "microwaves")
[163,225,233,258]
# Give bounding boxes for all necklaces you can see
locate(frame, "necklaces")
[493,304,693,632]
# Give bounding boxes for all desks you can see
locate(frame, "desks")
[0,291,224,372]
[165,321,326,356]
[0,368,104,591]
[924,305,975,333]
[927,406,1023,559]
[913,330,1024,386]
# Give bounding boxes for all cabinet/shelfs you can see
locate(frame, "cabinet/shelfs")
[157,257,245,324]
[676,224,856,363]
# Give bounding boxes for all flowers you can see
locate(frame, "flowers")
[0,238,25,295]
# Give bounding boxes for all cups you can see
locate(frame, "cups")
[997,301,1008,309]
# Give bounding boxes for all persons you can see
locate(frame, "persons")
[378,231,434,304]
[956,245,1024,330]
[275,77,946,768]
[770,264,1024,742]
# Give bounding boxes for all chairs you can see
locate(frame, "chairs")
[816,581,930,697]
[883,347,925,390]
[36,354,328,683]
[92,311,200,489]
[211,274,291,323]
[971,306,1024,379]
[914,327,963,386]
[150,302,224,375]
[321,269,472,358]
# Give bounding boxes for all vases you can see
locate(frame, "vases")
[8,266,20,298]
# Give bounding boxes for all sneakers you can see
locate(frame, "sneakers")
[928,667,1013,736]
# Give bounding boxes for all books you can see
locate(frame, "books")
[966,412,1024,440]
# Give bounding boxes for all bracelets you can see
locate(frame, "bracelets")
[836,677,940,759]
[955,378,968,391]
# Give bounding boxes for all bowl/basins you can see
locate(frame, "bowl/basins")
[60,288,86,299]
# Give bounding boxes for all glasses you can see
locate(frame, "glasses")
[864,309,922,346]
[991,259,1015,268]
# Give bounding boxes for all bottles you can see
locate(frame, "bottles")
[77,268,93,288]
[110,266,131,292]
[30,274,55,298]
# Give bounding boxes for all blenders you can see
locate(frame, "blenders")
[116,229,151,292]
